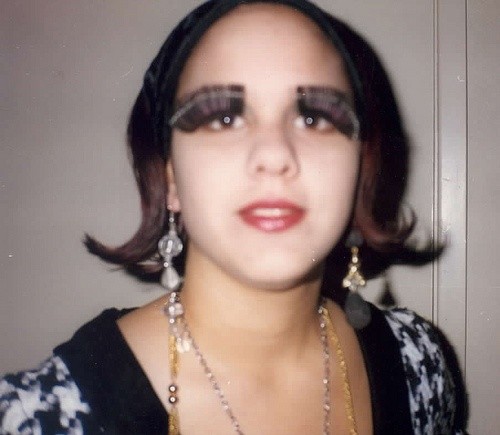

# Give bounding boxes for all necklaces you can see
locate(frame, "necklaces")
[176,295,336,435]
[161,290,361,434]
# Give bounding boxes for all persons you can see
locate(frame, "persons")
[1,0,471,435]
